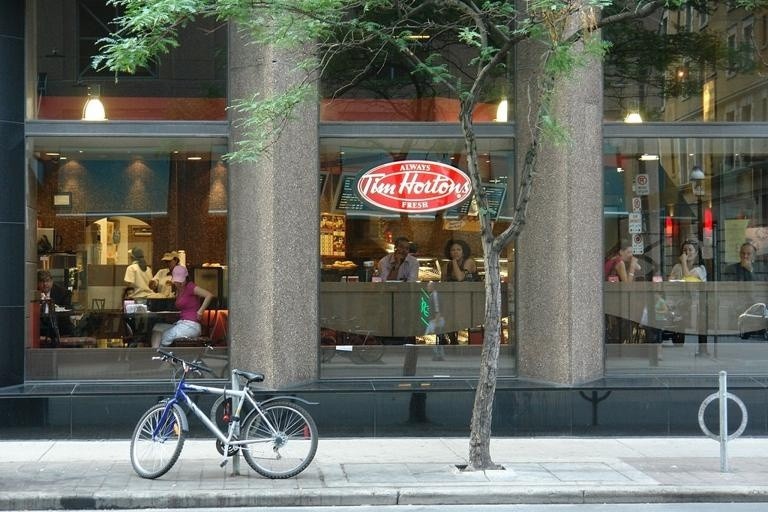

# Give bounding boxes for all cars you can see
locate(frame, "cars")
[657,300,684,343]
[416,258,442,281]
[738,303,768,340]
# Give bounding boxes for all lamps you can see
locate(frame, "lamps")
[689,165,704,180]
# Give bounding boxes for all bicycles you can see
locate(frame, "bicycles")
[129,348,320,479]
[317,328,384,364]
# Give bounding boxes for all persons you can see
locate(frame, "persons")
[148,251,182,296]
[121,247,156,300]
[601,238,641,284]
[723,242,765,281]
[654,292,669,361]
[423,281,447,361]
[150,265,215,348]
[125,287,135,297]
[668,239,707,282]
[36,270,68,313]
[437,238,479,346]
[377,236,417,283]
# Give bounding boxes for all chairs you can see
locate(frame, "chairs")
[40,297,221,348]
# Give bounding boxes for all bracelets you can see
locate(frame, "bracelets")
[197,312,203,315]
[628,272,634,276]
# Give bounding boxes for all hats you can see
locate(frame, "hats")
[171,265,188,284]
[128,247,145,260]
[161,248,180,262]
